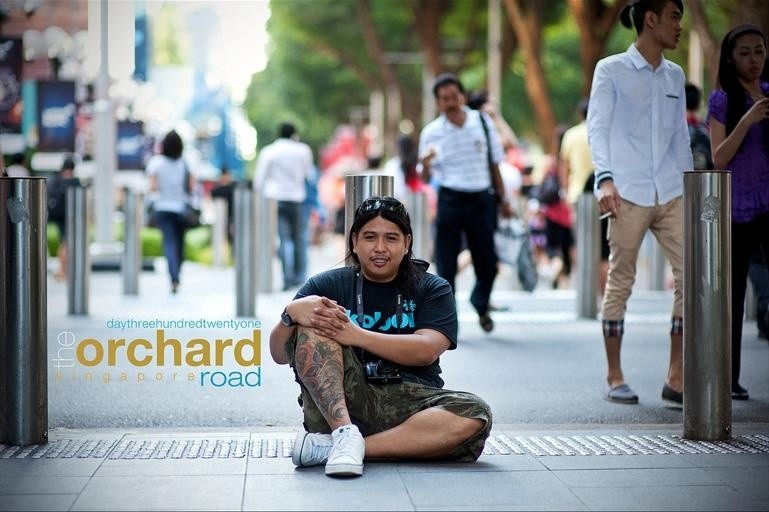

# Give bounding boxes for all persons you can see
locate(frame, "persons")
[50,74,712,333]
[270,195,493,476]
[706,24,769,401]
[586,0,695,404]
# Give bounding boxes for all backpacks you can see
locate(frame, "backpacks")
[690,123,715,170]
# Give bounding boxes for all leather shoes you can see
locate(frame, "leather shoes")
[601,383,639,404]
[472,301,493,331]
[661,382,683,405]
[732,385,749,401]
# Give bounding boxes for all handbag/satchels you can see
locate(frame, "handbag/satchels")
[182,204,202,227]
[517,242,546,293]
[478,110,507,199]
[537,171,559,204]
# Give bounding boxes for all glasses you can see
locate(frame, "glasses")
[356,199,408,217]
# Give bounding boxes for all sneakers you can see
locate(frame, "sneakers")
[324,423,367,477]
[291,430,332,468]
